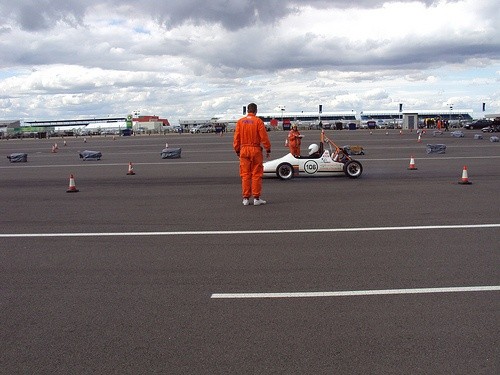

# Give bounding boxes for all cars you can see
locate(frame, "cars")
[263,148,363,180]
[0,126,182,139]
[308,119,403,131]
[419,117,500,133]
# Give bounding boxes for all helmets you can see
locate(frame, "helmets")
[292,123,298,130]
[308,144,318,155]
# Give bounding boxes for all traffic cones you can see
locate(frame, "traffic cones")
[458,165,473,185]
[406,154,418,170]
[51,145,56,153]
[83,136,87,143]
[126,161,135,175]
[284,138,289,147]
[63,139,67,147]
[417,135,422,143]
[54,142,59,150]
[65,173,79,192]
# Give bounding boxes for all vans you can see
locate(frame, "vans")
[189,125,210,134]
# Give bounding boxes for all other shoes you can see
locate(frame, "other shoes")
[254,198,266,206]
[243,198,250,205]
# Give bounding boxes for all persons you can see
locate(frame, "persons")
[424,115,450,131]
[233,103,271,206]
[289,123,302,156]
